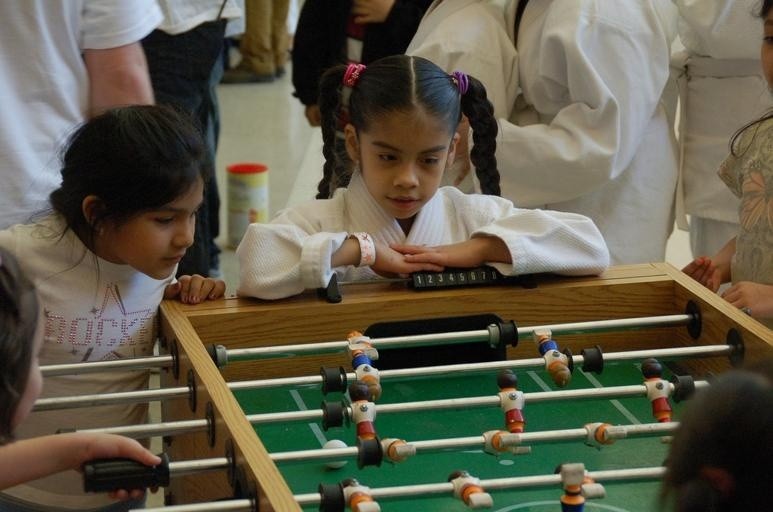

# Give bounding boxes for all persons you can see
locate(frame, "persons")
[653,367,773,512]
[0,101,234,510]
[681,0,773,326]
[131,0,249,287]
[663,2,772,262]
[229,51,614,303]
[289,0,539,194]
[214,2,293,87]
[0,261,170,510]
[456,0,681,265]
[0,0,166,233]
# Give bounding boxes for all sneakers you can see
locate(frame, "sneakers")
[218,67,284,83]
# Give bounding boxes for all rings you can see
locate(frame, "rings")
[741,307,751,316]
